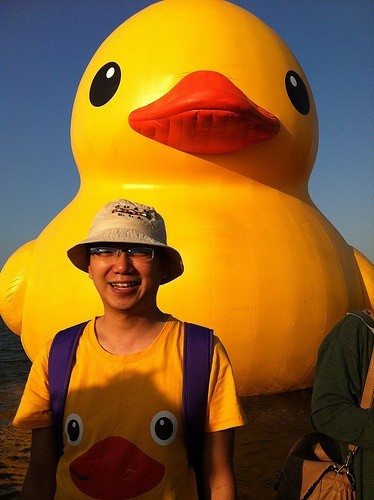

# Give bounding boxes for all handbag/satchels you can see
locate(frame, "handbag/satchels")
[276,455,357,500]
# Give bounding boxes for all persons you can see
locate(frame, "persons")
[311,310,374,500]
[11,200,248,500]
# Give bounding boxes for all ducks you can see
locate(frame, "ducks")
[1,0,374,398]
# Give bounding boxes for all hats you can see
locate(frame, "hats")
[67,199,184,285]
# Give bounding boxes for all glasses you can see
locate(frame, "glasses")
[89,247,162,262]
